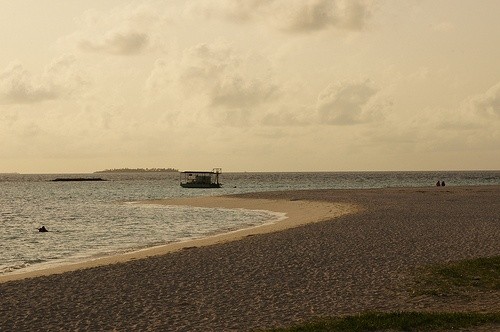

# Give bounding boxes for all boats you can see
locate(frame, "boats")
[36,227,48,232]
[45,176,111,182]
[179,168,224,188]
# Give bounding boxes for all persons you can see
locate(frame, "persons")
[436,181,440,187]
[441,180,445,186]
